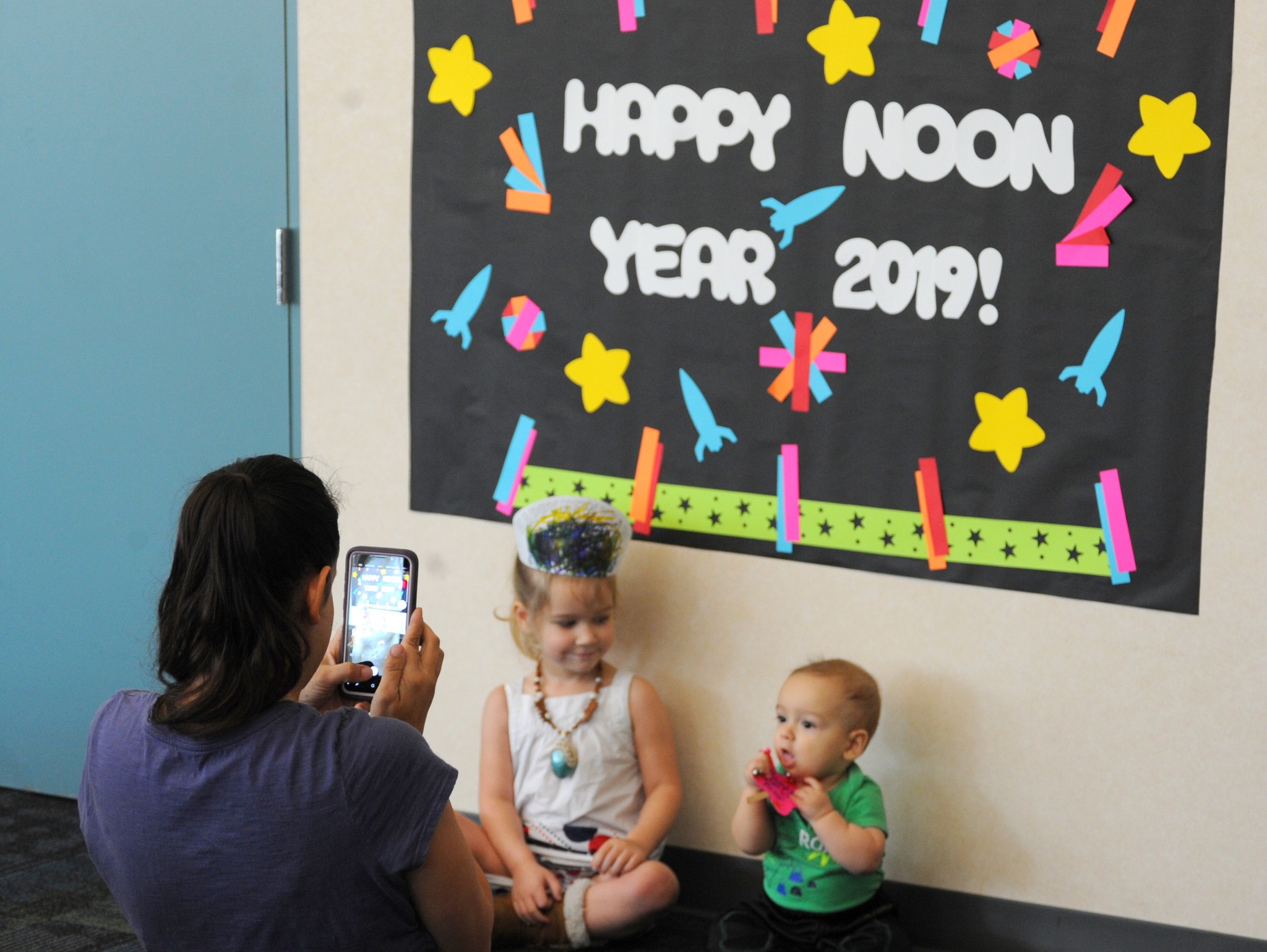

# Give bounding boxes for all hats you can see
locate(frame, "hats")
[511,498,635,579]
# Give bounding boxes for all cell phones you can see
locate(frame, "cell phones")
[338,546,419,701]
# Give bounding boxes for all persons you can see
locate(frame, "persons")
[454,493,682,952]
[705,659,910,952]
[80,453,494,952]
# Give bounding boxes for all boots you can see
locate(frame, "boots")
[483,876,591,952]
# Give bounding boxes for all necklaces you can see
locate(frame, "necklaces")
[533,659,603,779]
